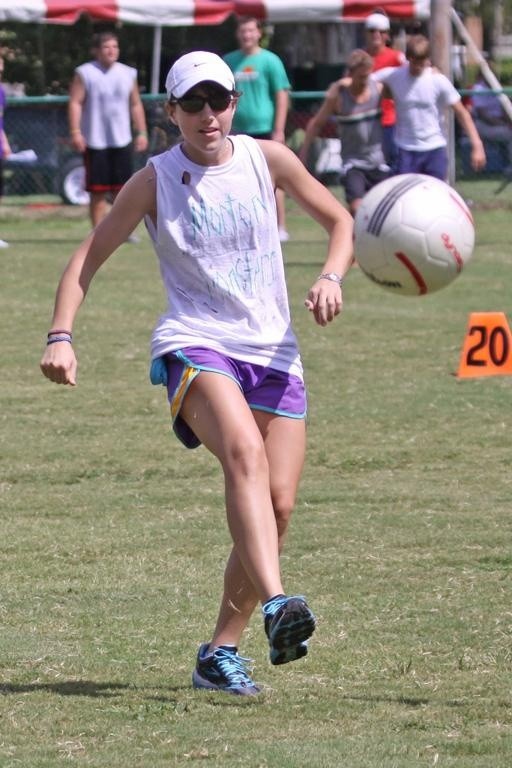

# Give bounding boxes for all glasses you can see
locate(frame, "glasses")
[168,92,234,113]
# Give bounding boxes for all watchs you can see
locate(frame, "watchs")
[316,271,344,285]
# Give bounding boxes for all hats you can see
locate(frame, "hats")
[363,12,390,32]
[165,50,237,101]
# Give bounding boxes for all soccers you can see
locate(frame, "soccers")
[352,173,475,296]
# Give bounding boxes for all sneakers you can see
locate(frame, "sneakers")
[192,640,263,700]
[263,591,318,665]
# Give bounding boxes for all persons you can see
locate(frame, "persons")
[35,49,355,699]
[221,14,292,244]
[0,53,17,250]
[324,32,487,180]
[67,31,149,246]
[297,47,441,223]
[362,13,406,167]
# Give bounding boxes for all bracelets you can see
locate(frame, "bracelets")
[48,329,73,337]
[69,128,82,133]
[133,130,147,136]
[46,336,72,344]
